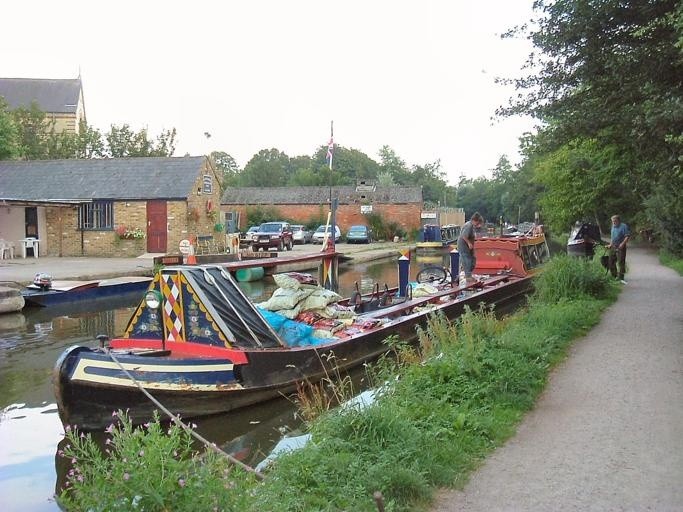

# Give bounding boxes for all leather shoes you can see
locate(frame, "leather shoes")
[466,277,477,282]
[615,275,624,281]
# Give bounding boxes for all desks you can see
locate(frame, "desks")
[19,240,42,259]
[206,240,225,255]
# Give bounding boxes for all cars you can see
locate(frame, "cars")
[245,226,260,245]
[346,225,373,244]
[290,225,312,244]
[313,224,341,244]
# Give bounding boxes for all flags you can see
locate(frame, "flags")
[326,125,335,172]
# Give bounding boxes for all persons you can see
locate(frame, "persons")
[605,214,630,282]
[457,211,483,285]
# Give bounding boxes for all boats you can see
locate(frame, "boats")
[415,223,462,248]
[53,212,565,431]
[567,221,595,256]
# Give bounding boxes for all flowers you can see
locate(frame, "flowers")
[112,225,127,236]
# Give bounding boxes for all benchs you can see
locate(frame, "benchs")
[195,232,220,255]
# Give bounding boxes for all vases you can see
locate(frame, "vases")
[120,235,124,239]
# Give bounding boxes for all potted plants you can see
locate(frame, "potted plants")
[124,225,146,239]
[152,260,166,276]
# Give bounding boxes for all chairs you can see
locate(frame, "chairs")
[0,239,15,261]
[24,238,37,259]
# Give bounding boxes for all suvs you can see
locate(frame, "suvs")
[251,222,294,252]
